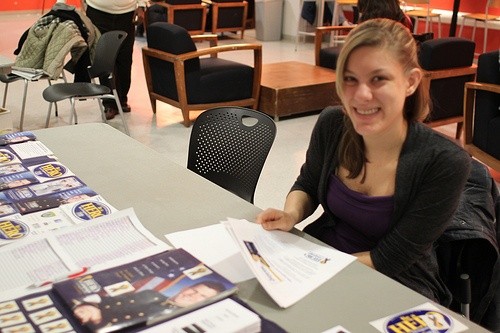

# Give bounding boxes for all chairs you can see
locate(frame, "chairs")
[42,31,130,137]
[458,0,500,54]
[143,0,207,42]
[187,106,277,203]
[462,51,500,175]
[0,62,78,131]
[295,0,360,48]
[142,23,262,126]
[403,0,441,38]
[314,16,478,139]
[201,0,253,39]
[435,159,500,329]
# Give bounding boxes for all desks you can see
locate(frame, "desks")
[0,123,491,333]
[259,60,342,121]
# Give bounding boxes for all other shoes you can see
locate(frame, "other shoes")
[121,101,131,112]
[104,102,119,120]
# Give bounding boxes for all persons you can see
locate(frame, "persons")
[352,0,407,27]
[256,17,500,333]
[70,282,227,333]
[80,0,150,122]
